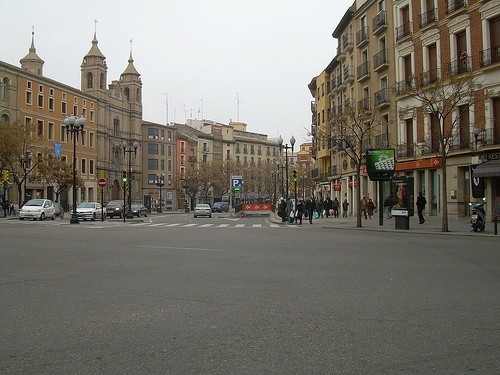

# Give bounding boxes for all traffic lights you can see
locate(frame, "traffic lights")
[4,170,10,181]
[122,170,127,182]
[293,170,297,182]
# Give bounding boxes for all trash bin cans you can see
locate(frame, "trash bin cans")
[391,209,414,230]
[187,207,189,213]
[158,207,160,213]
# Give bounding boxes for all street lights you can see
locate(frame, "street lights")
[155,173,164,213]
[271,134,296,200]
[123,139,138,219]
[64,113,87,224]
[183,175,191,213]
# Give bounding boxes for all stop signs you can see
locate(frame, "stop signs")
[98,179,107,187]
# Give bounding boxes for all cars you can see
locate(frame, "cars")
[106,199,129,218]
[211,202,229,212]
[69,202,107,221]
[19,199,63,221]
[131,204,148,217]
[192,203,212,218]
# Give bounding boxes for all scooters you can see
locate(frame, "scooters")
[468,197,486,232]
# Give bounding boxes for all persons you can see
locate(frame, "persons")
[4,200,15,215]
[384,195,393,220]
[333,197,340,218]
[360,195,367,219]
[415,192,427,224]
[343,199,349,218]
[366,198,376,219]
[276,194,332,225]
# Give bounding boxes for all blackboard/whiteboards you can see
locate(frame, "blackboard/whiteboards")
[493,199,500,216]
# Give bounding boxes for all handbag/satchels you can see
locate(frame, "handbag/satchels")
[329,208,334,215]
[304,210,308,217]
[323,210,325,215]
[295,210,298,216]
[290,210,295,217]
[315,212,319,220]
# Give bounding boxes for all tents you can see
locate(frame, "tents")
[473,161,500,177]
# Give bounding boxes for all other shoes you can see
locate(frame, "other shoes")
[419,219,425,225]
